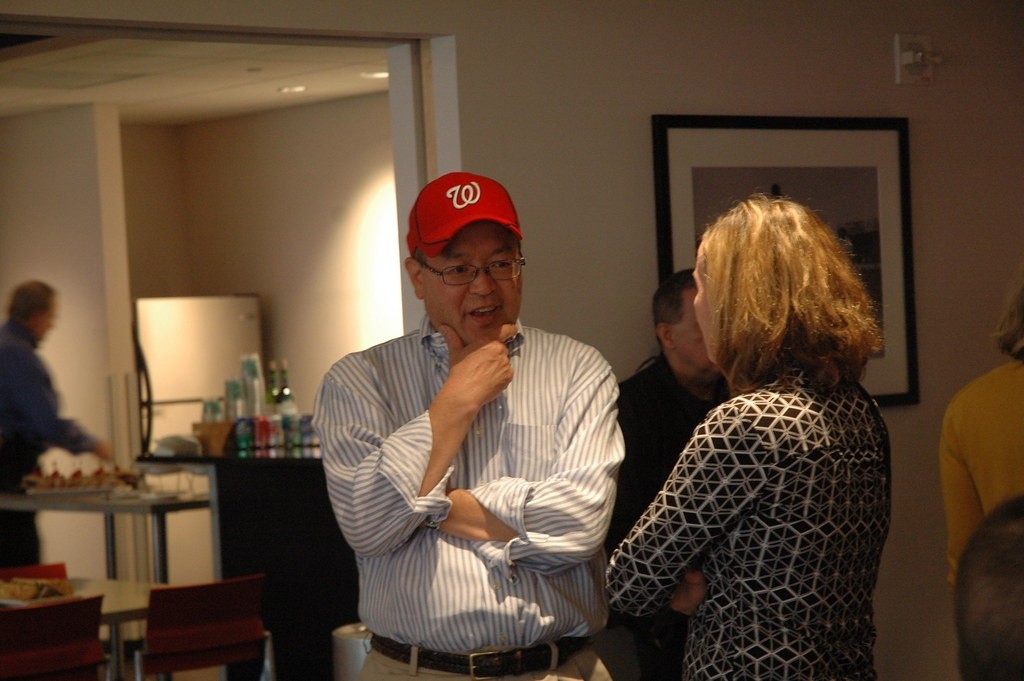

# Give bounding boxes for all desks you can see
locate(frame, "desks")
[0,577,151,681]
[0,493,211,681]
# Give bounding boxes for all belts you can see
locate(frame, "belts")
[370,633,591,678]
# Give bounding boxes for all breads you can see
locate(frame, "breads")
[0,578,74,602]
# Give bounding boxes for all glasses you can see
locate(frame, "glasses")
[420,250,526,285]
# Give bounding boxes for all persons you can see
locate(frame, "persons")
[606,196,891,681]
[954,495,1024,681]
[312,172,626,681]
[939,270,1024,599]
[602,268,729,681]
[0,281,111,572]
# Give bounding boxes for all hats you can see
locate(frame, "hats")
[406,172,523,258]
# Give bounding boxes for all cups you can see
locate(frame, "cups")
[201,353,266,423]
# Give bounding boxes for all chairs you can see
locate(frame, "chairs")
[134,573,273,681]
[0,563,68,585]
[0,593,107,681]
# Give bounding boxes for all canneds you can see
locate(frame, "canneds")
[235,414,320,450]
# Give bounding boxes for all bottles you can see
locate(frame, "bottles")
[274,360,298,416]
[266,360,285,415]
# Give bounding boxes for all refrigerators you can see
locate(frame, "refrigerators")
[135,298,261,585]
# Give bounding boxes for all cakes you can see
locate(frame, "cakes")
[26,465,143,494]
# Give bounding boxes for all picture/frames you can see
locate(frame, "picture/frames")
[652,114,921,409]
